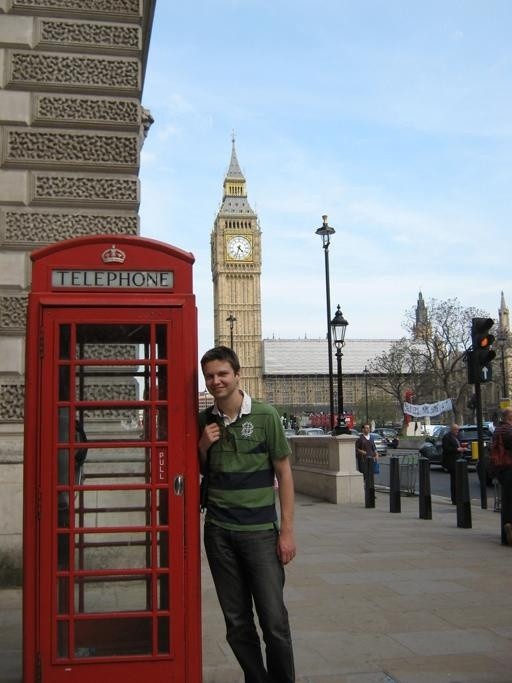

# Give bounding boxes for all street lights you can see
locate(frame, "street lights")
[226,314,236,350]
[315,215,335,431]
[363,365,370,424]
[330,304,352,434]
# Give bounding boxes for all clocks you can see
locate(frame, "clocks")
[227,234,252,260]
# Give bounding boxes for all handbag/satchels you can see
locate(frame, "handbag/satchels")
[358,456,365,473]
[374,462,380,473]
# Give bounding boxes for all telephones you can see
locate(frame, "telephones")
[58,406,88,516]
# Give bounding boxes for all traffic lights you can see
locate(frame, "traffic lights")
[472,317,497,363]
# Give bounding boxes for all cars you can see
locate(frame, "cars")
[420,425,492,471]
[350,427,399,457]
[283,428,324,435]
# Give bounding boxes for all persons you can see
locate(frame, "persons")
[280,412,289,429]
[198,344,297,683]
[356,423,379,499]
[391,435,398,449]
[491,406,511,548]
[442,422,467,505]
[289,412,303,434]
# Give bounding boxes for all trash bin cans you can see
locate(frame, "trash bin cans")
[392,450,422,497]
